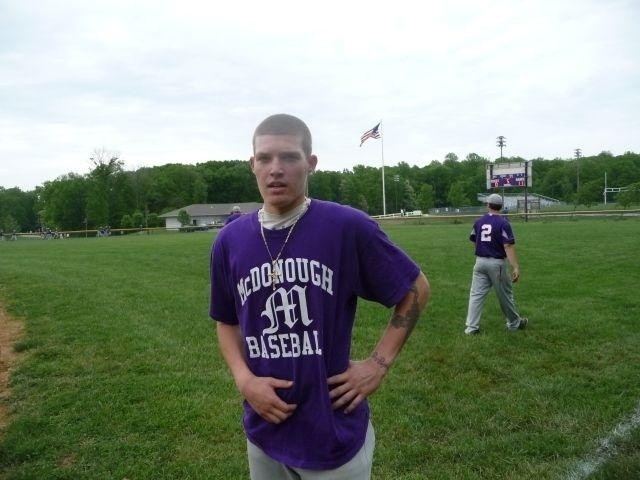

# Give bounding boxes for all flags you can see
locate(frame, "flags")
[359,122,381,148]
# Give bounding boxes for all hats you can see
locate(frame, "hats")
[484,194,506,206]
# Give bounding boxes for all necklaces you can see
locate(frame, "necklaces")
[260,194,309,291]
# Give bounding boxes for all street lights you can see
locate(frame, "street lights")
[110,184,118,232]
[495,135,506,210]
[37,192,44,234]
[392,173,399,212]
[574,147,583,193]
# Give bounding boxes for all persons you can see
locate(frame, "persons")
[11,230,17,240]
[209,115,430,479]
[225,205,246,226]
[39,226,59,240]
[400,208,404,217]
[98,226,112,238]
[503,207,511,222]
[464,193,528,334]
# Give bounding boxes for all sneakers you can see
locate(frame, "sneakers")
[463,325,483,336]
[508,318,533,331]
